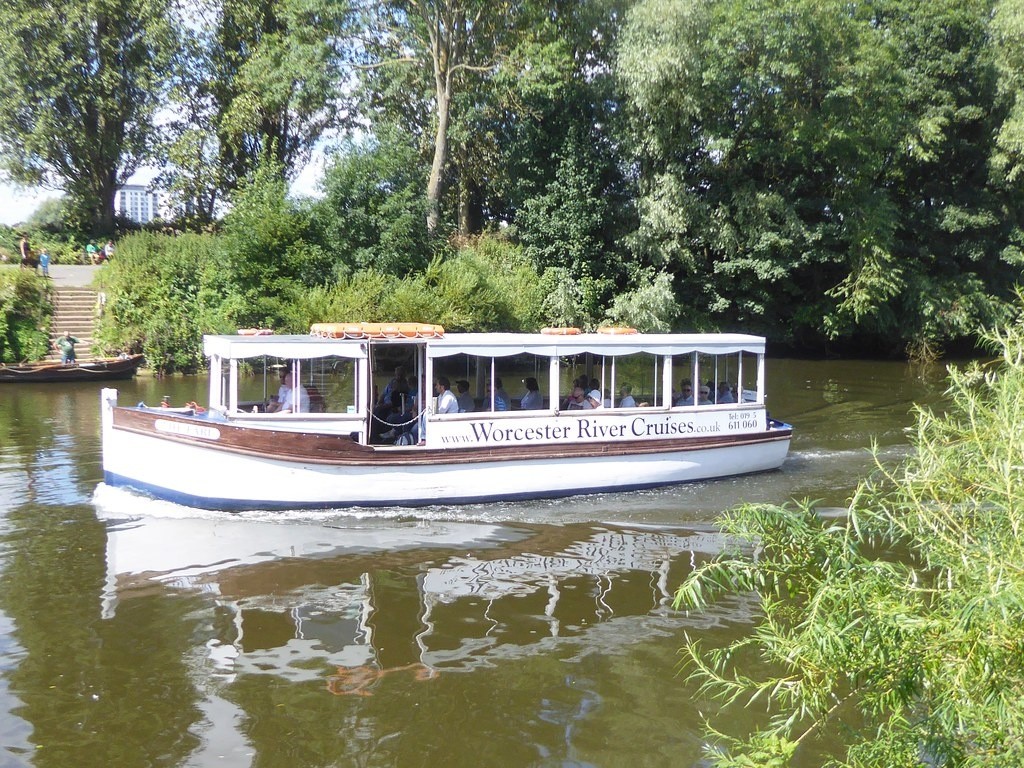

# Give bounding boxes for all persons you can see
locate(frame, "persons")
[20,231,115,280]
[271,367,744,445]
[53,330,91,364]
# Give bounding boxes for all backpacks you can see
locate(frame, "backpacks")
[395,432,414,445]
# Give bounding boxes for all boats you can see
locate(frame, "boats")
[98,325,796,515]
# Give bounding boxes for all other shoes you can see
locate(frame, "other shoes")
[417,441,426,446]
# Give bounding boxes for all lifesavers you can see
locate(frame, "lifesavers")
[596,326,638,335]
[237,328,275,336]
[540,327,581,335]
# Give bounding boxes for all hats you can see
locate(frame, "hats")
[573,388,585,397]
[455,380,469,388]
[90,239,97,244]
[588,389,601,404]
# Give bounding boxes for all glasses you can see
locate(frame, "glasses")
[573,397,579,399]
[683,389,691,392]
[700,391,708,395]
[587,396,592,399]
